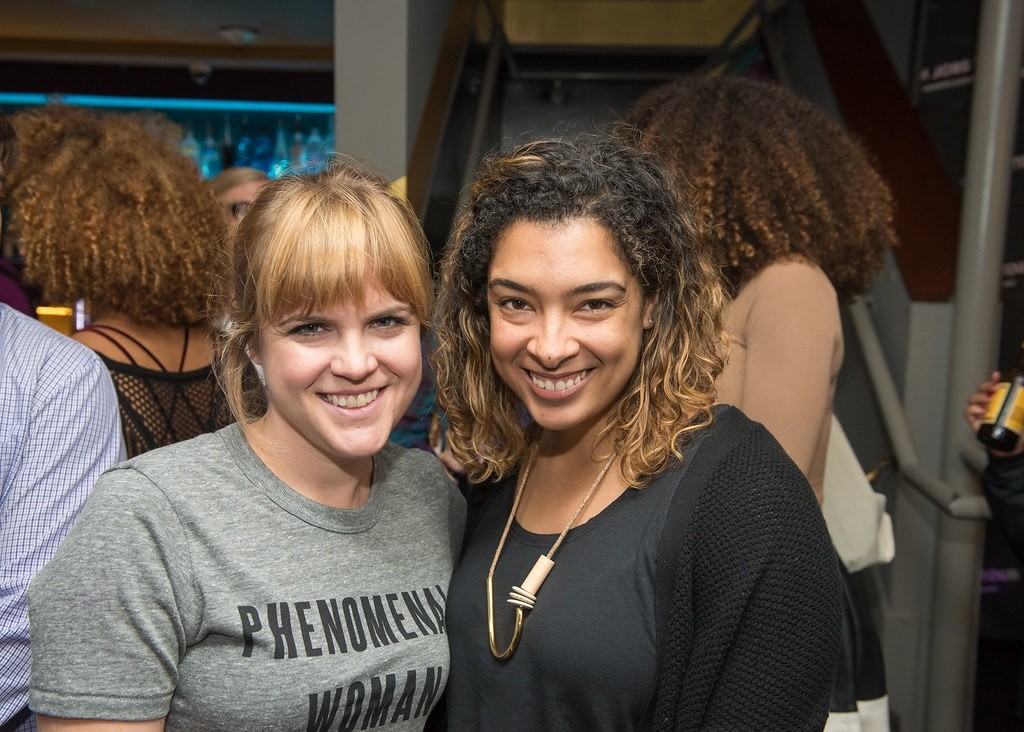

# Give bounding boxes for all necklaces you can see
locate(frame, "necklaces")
[486,435,624,659]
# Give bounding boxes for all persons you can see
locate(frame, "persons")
[964,371,1024,565]
[0,74,894,732]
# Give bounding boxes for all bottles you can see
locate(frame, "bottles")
[977,341,1024,453]
[183,125,337,179]
